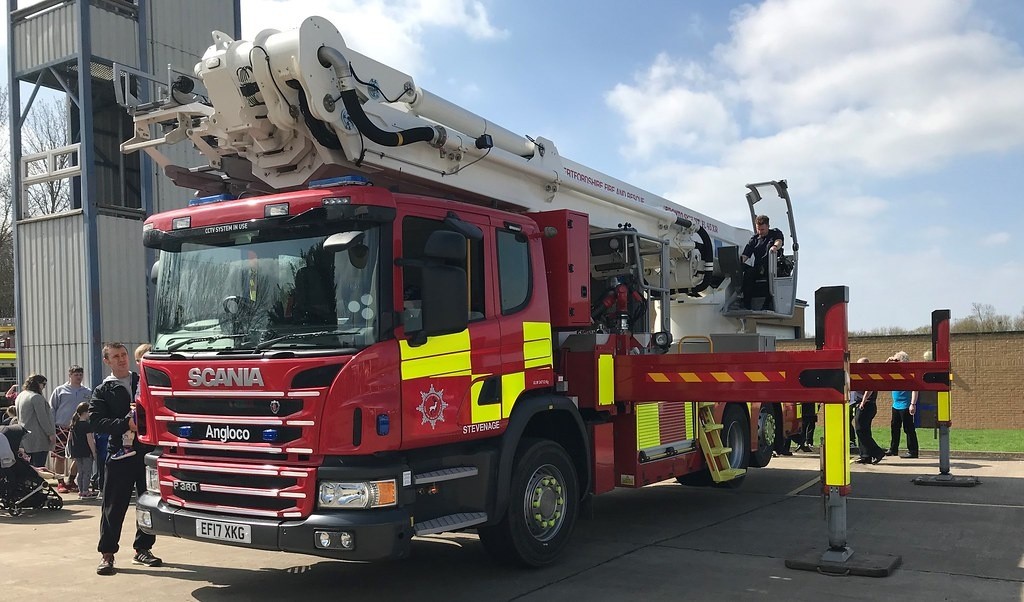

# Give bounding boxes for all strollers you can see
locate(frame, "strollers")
[0,424,63,516]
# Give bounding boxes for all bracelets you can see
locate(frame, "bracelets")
[773,245,777,247]
[911,403,916,406]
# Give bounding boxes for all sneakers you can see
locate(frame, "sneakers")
[111,447,137,460]
[57,483,68,493]
[81,491,97,499]
[97,553,114,574]
[68,482,78,491]
[78,492,81,499]
[132,549,162,566]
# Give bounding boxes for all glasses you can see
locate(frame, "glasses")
[41,382,46,386]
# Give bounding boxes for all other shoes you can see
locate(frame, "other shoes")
[855,459,868,463]
[871,457,881,463]
[900,453,918,458]
[885,450,898,455]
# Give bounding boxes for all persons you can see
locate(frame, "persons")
[49,365,93,493]
[89,342,162,575]
[885,351,919,458]
[71,402,108,500]
[849,358,886,465]
[110,344,152,461]
[15,373,56,466]
[796,401,821,452]
[6,383,18,425]
[740,215,784,311]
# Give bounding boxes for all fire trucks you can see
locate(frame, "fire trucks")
[113,15,983,578]
